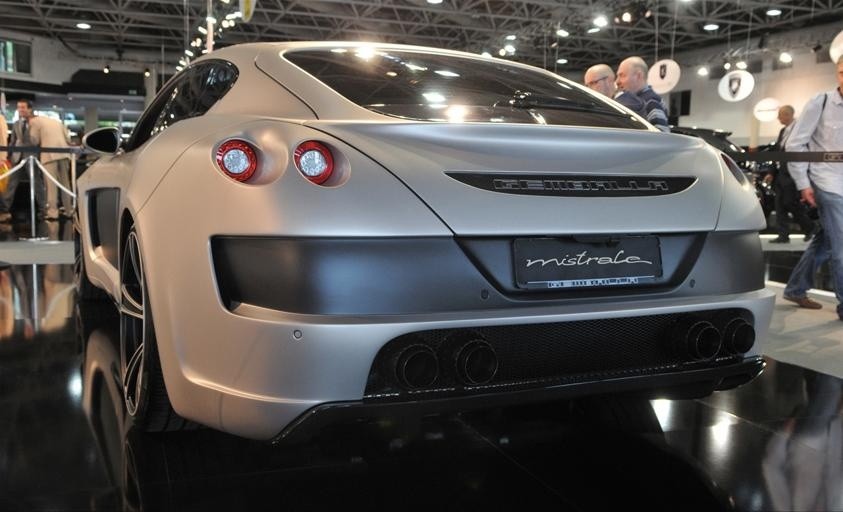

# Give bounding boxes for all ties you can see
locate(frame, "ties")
[21,120,27,136]
[776,128,785,142]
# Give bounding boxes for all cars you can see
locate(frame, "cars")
[673,122,766,214]
[740,140,817,228]
[71,39,779,450]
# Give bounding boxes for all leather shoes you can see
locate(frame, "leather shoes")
[783,293,822,309]
[803,222,817,241]
[0,212,12,221]
[769,236,789,244]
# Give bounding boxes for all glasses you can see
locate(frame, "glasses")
[585,76,607,87]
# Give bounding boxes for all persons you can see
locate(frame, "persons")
[613,55,671,133]
[780,54,843,321]
[758,360,842,512]
[762,105,816,244]
[584,61,649,124]
[0,98,89,227]
[0,220,80,347]
